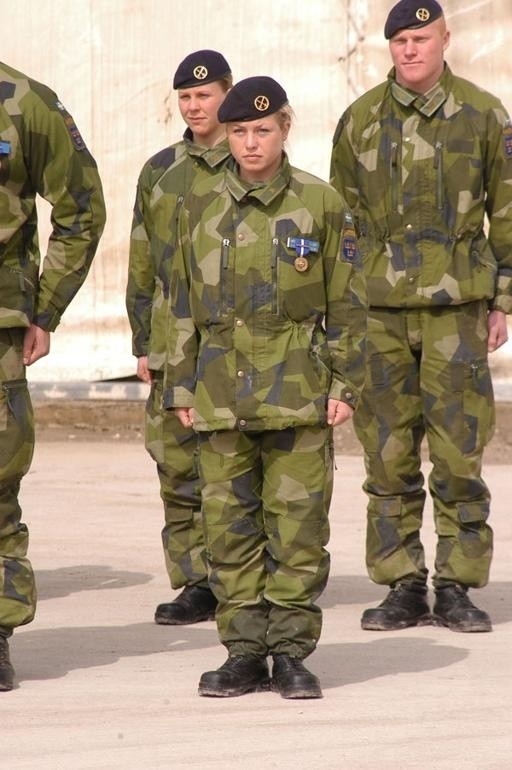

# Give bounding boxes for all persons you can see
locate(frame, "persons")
[123,47,237,630]
[161,75,370,702]
[1,60,109,692]
[326,0,512,636]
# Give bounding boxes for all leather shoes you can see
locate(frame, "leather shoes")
[198,653,322,698]
[0,638,15,689]
[361,584,492,632]
[155,586,219,625]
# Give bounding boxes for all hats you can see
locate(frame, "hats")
[173,49,230,90]
[384,0,443,40]
[217,76,289,123]
